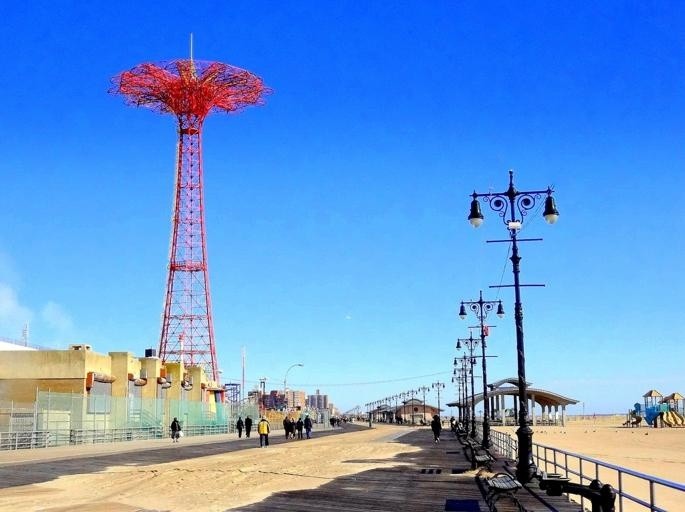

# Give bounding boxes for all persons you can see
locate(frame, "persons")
[281,413,314,441]
[169,416,182,444]
[430,414,443,444]
[257,415,270,448]
[244,415,254,438]
[450,415,457,432]
[236,416,244,438]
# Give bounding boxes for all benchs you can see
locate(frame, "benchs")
[450,416,528,512]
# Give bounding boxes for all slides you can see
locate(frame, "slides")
[631,410,642,425]
[663,410,685,427]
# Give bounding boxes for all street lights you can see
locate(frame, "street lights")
[451,169,560,483]
[364,380,446,425]
[284,362,305,389]
